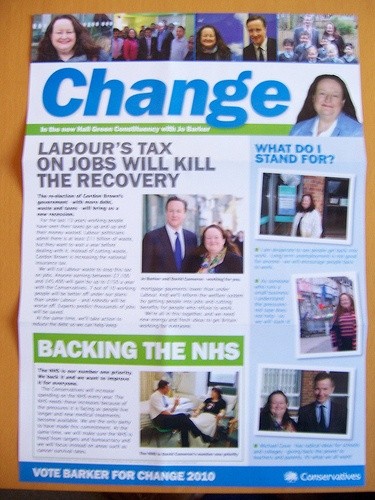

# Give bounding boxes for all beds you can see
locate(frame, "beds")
[141,389,196,437]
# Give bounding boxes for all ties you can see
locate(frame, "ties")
[258,47,265,61]
[319,405,326,431]
[162,396,168,407]
[175,232,182,272]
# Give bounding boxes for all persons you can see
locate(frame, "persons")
[298,372,348,434]
[196,25,232,61]
[223,399,238,447]
[112,18,193,60]
[243,15,277,60]
[36,14,110,61]
[178,387,227,447]
[149,380,219,447]
[142,197,197,273]
[330,291,357,352]
[287,74,364,137]
[186,224,244,273]
[278,14,359,64]
[291,193,323,239]
[260,390,302,432]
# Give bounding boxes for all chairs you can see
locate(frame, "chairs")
[181,386,238,448]
[146,411,174,447]
